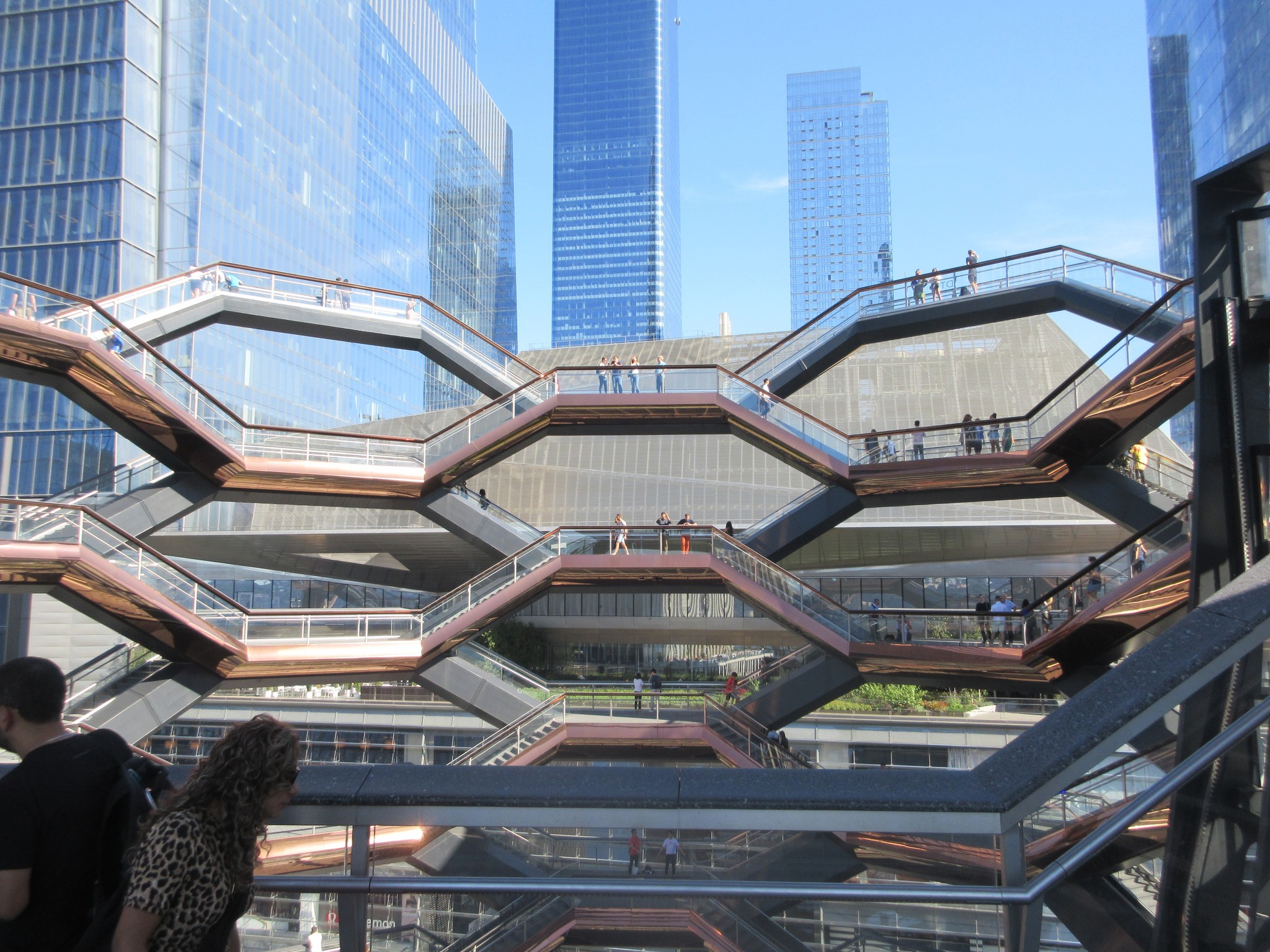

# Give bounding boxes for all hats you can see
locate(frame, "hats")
[651,668,656,672]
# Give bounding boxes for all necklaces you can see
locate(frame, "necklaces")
[30,731,78,750]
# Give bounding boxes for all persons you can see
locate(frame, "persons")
[723,660,770,708]
[612,511,733,556]
[320,277,351,310]
[405,298,419,321]
[911,250,979,304]
[759,379,772,420]
[0,656,302,952]
[190,264,244,298]
[1111,440,1148,485]
[596,355,667,394]
[869,599,913,644]
[308,926,322,952]
[666,652,705,660]
[11,284,37,321]
[864,413,1012,465]
[634,668,662,712]
[976,538,1146,647]
[101,324,124,363]
[628,829,685,879]
[552,370,560,398]
[461,481,491,511]
[1175,491,1193,542]
[880,763,886,769]
[763,724,788,769]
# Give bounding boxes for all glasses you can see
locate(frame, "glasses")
[282,768,299,785]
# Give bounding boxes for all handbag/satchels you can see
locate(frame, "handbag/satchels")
[959,286,971,296]
[884,634,895,643]
[628,867,639,876]
[929,283,939,289]
[72,882,118,951]
[482,498,491,506]
[627,370,632,378]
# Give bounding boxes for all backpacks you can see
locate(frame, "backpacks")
[86,756,173,916]
[596,362,603,374]
[654,677,662,688]
